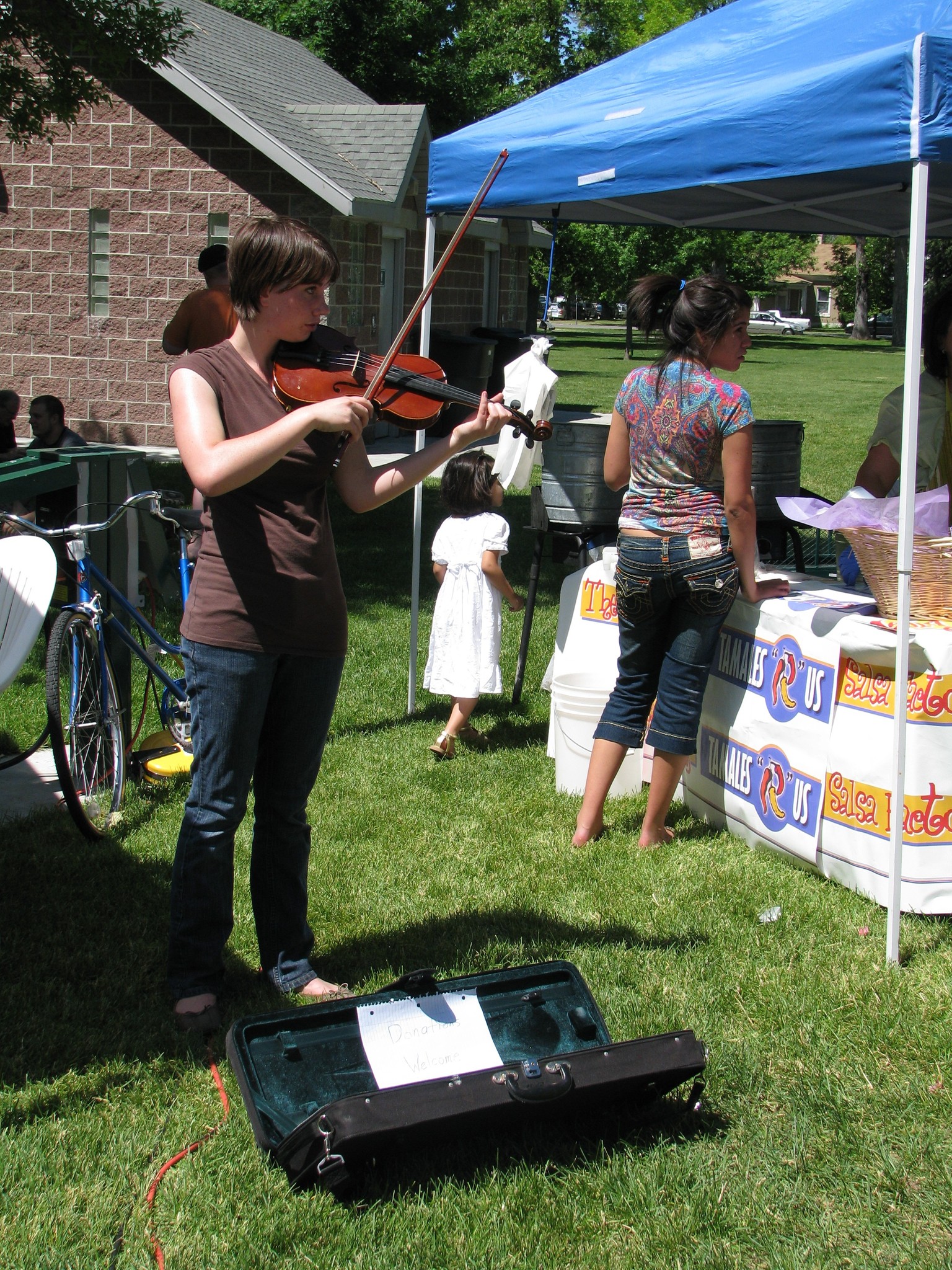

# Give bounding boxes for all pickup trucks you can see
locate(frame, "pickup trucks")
[762,310,812,330]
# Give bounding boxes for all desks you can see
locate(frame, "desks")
[540,560,952,915]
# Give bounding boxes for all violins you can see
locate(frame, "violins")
[272,324,554,449]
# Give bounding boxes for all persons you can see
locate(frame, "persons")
[853,295,952,527]
[161,216,514,1018]
[423,447,527,756]
[568,269,792,850]
[0,390,88,579]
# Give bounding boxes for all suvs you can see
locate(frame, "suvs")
[844,312,893,336]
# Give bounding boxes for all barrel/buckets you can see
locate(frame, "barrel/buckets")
[541,421,629,526]
[751,420,805,520]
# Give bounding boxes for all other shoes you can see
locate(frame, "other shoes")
[173,1002,219,1033]
[296,981,355,1000]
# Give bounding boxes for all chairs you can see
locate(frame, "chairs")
[0,535,57,690]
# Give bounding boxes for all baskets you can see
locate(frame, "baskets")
[841,526,952,620]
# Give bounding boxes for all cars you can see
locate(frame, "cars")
[746,311,805,335]
[537,295,627,321]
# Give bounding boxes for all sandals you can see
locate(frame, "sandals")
[459,724,490,746]
[429,731,457,759]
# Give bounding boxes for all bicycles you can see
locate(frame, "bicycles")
[0,486,204,844]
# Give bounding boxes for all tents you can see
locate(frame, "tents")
[405,0,952,960]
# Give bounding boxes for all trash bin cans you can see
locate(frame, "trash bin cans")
[409,328,498,438]
[470,326,523,340]
[496,333,556,404]
